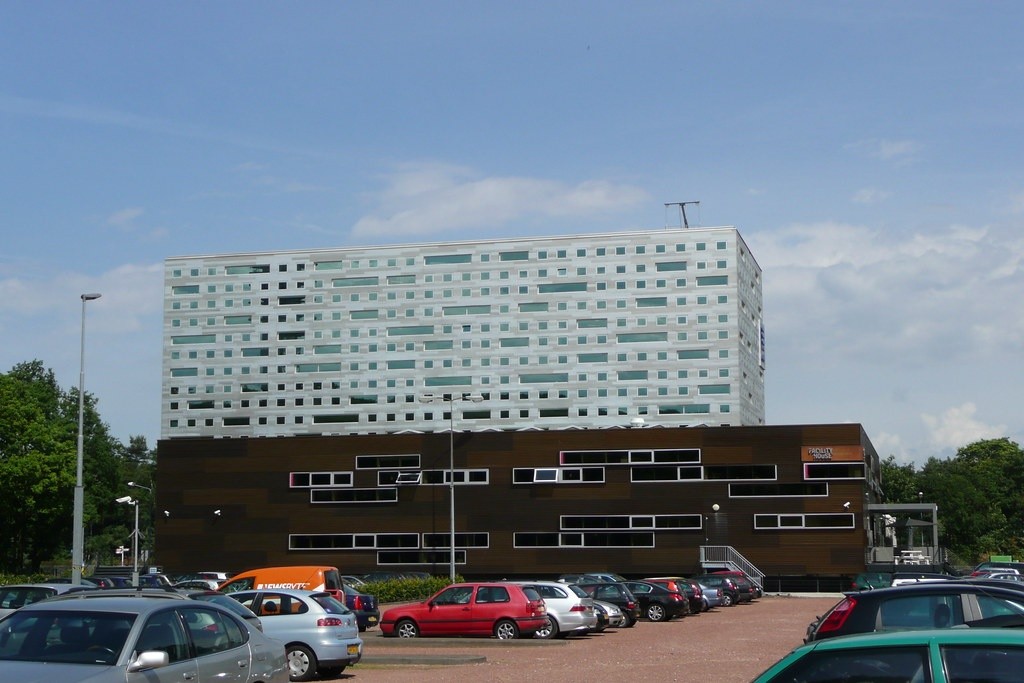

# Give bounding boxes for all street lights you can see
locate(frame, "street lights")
[419,394,484,585]
[127,481,153,557]
[72,293,103,585]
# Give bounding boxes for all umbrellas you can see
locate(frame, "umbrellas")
[883,517,937,550]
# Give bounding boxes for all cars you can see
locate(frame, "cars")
[341,576,366,589]
[892,568,1024,589]
[557,573,626,586]
[0,594,289,683]
[590,599,623,633]
[46,572,240,597]
[570,583,640,628]
[359,570,434,582]
[645,577,722,616]
[342,580,379,633]
[597,580,684,624]
[379,583,549,639]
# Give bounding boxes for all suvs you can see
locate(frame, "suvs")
[226,590,362,681]
[803,582,1024,635]
[699,572,753,607]
[59,585,264,636]
[459,579,598,639]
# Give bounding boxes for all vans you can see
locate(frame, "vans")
[974,562,1024,574]
[0,583,95,634]
[206,565,347,615]
[750,627,1024,683]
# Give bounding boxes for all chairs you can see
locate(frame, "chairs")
[476,589,496,603]
[975,650,1012,683]
[46,625,215,655]
[298,603,310,614]
[934,605,952,627]
[264,601,278,615]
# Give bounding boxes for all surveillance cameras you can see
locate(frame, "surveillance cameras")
[165,511,169,515]
[215,510,220,515]
[116,497,131,504]
[844,502,850,507]
